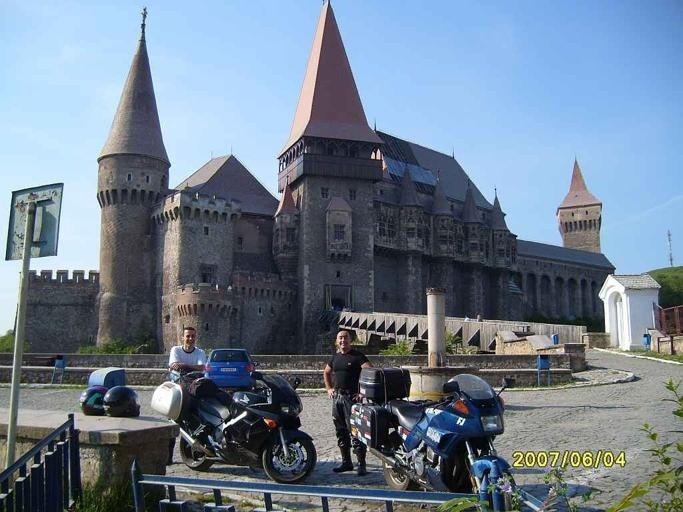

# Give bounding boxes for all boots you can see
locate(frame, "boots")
[356,453,367,475]
[333,447,353,472]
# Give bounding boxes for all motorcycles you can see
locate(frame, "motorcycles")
[169,369,317,484]
[349,366,516,512]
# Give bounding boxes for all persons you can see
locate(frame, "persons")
[323,328,373,477]
[169,326,206,387]
[477,314,483,322]
[464,313,471,321]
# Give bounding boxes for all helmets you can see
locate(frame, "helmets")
[78,386,109,416]
[102,385,140,417]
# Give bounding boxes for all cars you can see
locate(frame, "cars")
[203,349,256,392]
